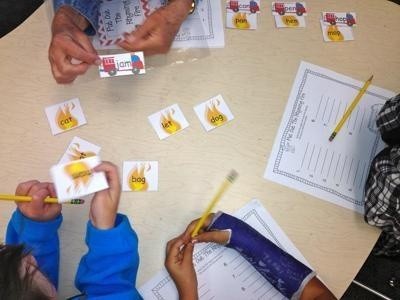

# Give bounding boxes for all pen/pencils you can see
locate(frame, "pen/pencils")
[190,169,240,239]
[0,194,85,205]
[329,74,374,144]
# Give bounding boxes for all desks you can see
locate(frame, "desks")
[1,1,400,299]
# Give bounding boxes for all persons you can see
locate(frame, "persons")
[48,0,200,84]
[361,92,399,257]
[0,161,144,300]
[165,210,337,299]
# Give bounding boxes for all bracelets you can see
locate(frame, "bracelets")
[189,1,195,15]
